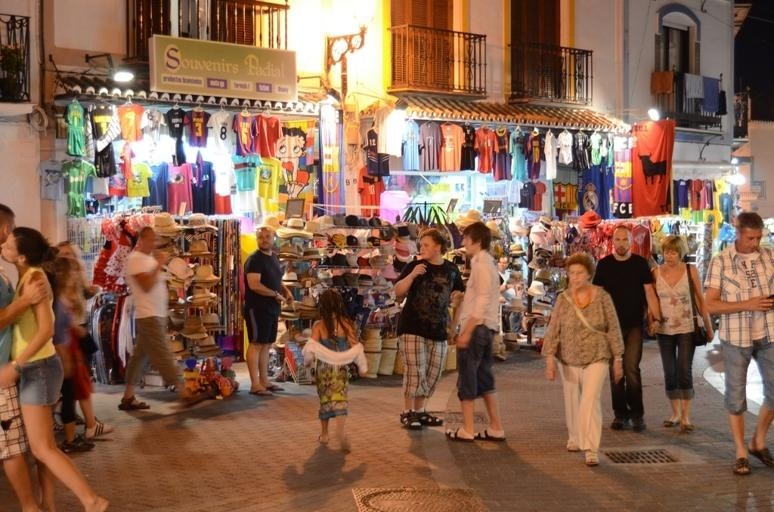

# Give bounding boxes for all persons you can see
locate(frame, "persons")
[1,227,111,511]
[496,252,525,334]
[303,287,369,450]
[274,127,308,201]
[238,257,246,319]
[53,257,96,454]
[540,252,626,465]
[57,240,97,426]
[703,211,774,476]
[646,235,715,434]
[242,226,295,397]
[444,222,506,442]
[117,226,185,411]
[0,202,49,511]
[592,224,663,431]
[40,247,60,309]
[394,229,465,430]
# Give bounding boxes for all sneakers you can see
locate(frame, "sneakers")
[53,423,62,431]
[611,418,628,429]
[60,434,95,454]
[632,419,645,431]
[566,439,579,451]
[584,451,600,465]
[85,422,113,437]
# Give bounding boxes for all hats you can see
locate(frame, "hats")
[331,232,394,247]
[374,299,400,315]
[506,270,525,285]
[539,216,552,231]
[151,212,177,236]
[187,239,215,256]
[282,272,302,289]
[159,257,194,279]
[178,212,218,232]
[577,209,601,231]
[534,269,552,283]
[187,283,217,303]
[169,340,190,356]
[334,212,390,227]
[168,290,184,310]
[373,276,391,290]
[508,244,527,256]
[275,215,336,240]
[298,296,316,311]
[280,300,300,317]
[202,313,225,332]
[327,252,387,267]
[192,264,220,282]
[526,280,546,297]
[377,265,400,280]
[278,246,322,263]
[181,318,208,339]
[193,336,220,353]
[456,209,502,240]
[325,271,373,287]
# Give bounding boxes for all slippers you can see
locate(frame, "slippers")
[248,389,272,396]
[267,385,285,392]
[748,447,773,468]
[732,457,751,475]
[475,429,506,442]
[84,497,109,511]
[445,428,475,442]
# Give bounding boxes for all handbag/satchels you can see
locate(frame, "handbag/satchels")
[686,263,708,346]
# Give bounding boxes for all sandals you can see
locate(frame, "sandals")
[399,411,422,430]
[663,415,681,426]
[417,411,443,427]
[117,395,150,410]
[681,422,694,434]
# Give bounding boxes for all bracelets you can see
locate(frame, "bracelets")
[614,357,623,362]
[274,290,280,298]
[653,319,661,324]
[11,360,23,374]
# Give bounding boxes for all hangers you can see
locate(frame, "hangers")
[463,121,471,127]
[172,100,181,110]
[497,124,507,130]
[194,101,204,111]
[481,122,492,131]
[559,127,626,138]
[532,127,539,134]
[216,103,227,112]
[242,105,250,114]
[545,128,556,136]
[92,92,105,101]
[406,116,414,122]
[72,94,80,102]
[123,95,135,105]
[426,118,436,125]
[261,108,272,115]
[46,146,59,162]
[513,125,524,132]
[442,118,453,126]
[147,99,157,108]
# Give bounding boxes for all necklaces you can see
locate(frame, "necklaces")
[571,284,593,309]
